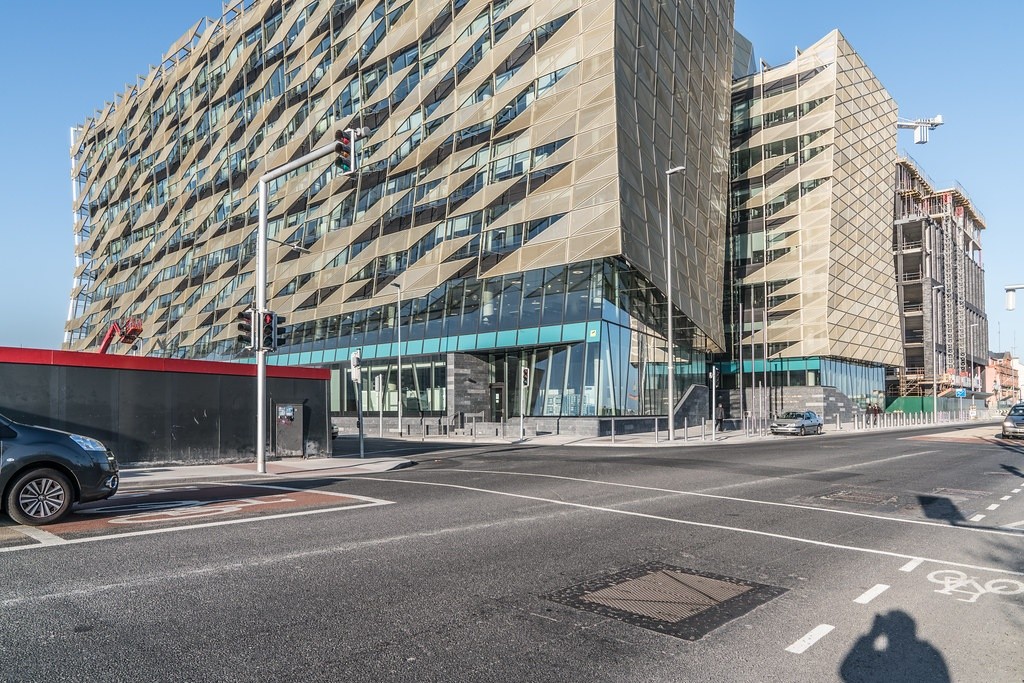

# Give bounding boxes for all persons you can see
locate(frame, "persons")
[866,403,883,425]
[715,403,725,431]
[602,406,608,416]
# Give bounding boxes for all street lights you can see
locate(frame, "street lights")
[389,282,402,439]
[256,232,310,476]
[664,166,687,440]
[970,323,980,411]
[931,284,945,423]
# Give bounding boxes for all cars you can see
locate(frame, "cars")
[770,409,824,436]
[1001,402,1024,440]
[0,414,120,526]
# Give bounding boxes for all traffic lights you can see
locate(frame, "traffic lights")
[334,128,354,175]
[274,314,286,351]
[259,311,274,351]
[522,367,529,387]
[237,309,254,349]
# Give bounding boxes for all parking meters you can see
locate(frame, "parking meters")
[350,348,365,459]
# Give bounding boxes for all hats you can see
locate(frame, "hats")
[869,404,871,406]
